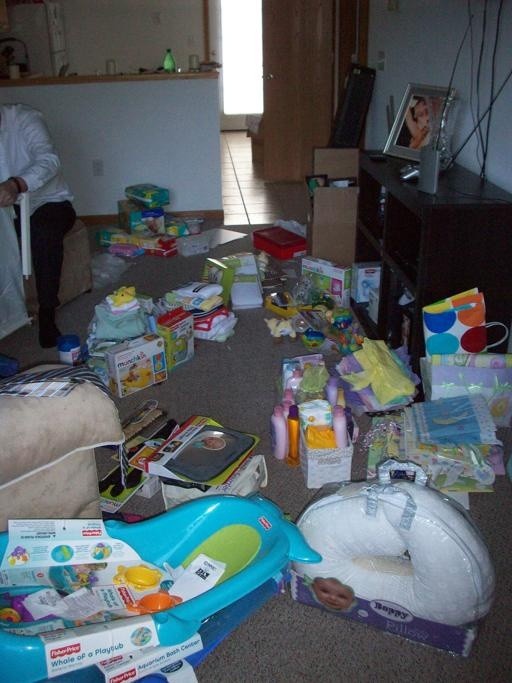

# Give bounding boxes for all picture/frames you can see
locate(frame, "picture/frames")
[382,81,457,167]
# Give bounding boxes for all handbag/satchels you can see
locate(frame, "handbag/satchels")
[420,351,512,427]
[422,288,508,354]
[161,455,266,510]
[290,462,492,656]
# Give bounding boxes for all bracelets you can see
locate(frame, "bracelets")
[7,176,22,194]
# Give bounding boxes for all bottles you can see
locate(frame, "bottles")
[163,48,176,74]
[55,334,83,367]
[269,361,355,462]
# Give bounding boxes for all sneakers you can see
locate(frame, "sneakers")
[39,324,61,349]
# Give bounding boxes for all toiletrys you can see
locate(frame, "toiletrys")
[268,357,350,467]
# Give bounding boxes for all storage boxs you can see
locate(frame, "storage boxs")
[304,143,361,268]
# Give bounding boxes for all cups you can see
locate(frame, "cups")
[8,64,21,80]
[105,60,116,75]
[188,54,200,73]
[425,93,460,170]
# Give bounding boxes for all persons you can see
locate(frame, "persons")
[313,576,354,610]
[1,99,78,352]
[404,92,431,149]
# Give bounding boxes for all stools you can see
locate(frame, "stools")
[15,217,94,319]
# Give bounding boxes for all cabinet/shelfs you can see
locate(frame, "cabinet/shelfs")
[350,148,510,392]
